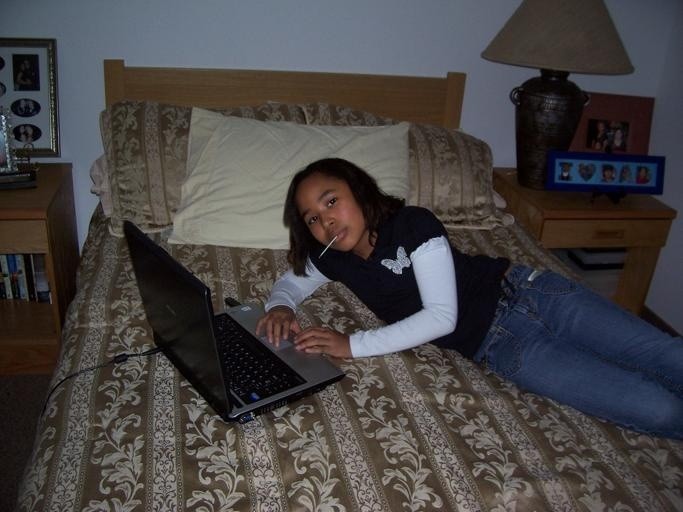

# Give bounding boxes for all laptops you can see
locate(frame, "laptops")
[123,219,346,425]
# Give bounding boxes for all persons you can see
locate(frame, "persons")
[254,156,683,441]
[558,160,653,185]
[17,59,36,90]
[592,120,626,152]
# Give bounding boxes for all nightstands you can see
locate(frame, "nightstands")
[0,162,81,375]
[493,166,677,318]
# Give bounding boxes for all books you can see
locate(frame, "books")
[0,254,53,304]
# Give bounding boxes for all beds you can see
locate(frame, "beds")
[13,59,683,512]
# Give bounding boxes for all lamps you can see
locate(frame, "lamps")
[481,0,634,191]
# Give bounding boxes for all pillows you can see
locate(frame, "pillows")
[298,101,493,229]
[98,100,307,236]
[168,107,410,250]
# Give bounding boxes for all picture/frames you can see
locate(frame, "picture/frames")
[0,38,61,158]
[548,150,665,195]
[569,92,655,155]
